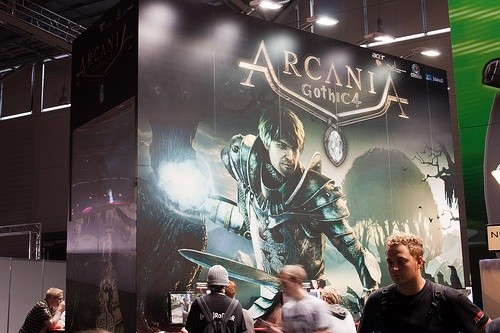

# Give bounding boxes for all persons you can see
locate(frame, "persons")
[356,233,494,333]
[260,265,334,333]
[222,279,256,332]
[179,265,248,333]
[316,284,357,333]
[219,108,382,325]
[17,287,66,333]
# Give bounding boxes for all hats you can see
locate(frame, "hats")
[206,264,229,285]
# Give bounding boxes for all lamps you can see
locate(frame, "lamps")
[243,0,280,16]
[359,32,391,46]
[358,0,450,47]
[297,15,339,29]
[402,47,441,60]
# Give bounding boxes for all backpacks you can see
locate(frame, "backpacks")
[196,296,240,333]
